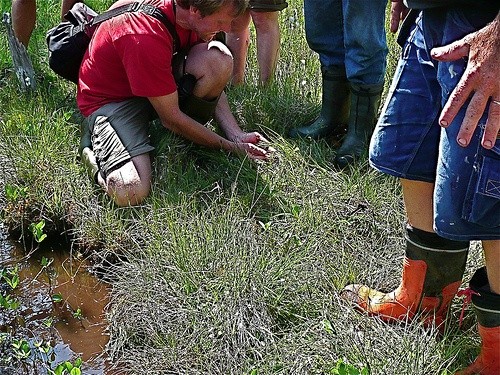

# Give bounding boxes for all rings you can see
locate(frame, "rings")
[493,100,500,105]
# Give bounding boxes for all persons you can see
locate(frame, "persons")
[78,0,283,204]
[225,0,288,90]
[12,0,84,50]
[338,0,500,375]
[289,0,388,170]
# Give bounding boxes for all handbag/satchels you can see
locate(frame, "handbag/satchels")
[44,3,99,84]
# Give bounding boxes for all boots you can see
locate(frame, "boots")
[79,145,100,183]
[339,232,470,339]
[288,68,348,141]
[177,73,227,174]
[451,266,500,374]
[333,84,385,172]
